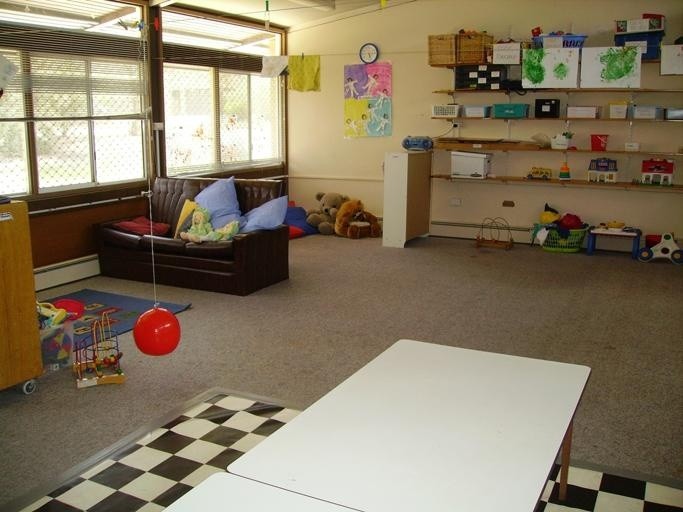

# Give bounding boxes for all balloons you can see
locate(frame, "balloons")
[132,304,180,356]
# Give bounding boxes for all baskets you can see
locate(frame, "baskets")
[533,220,589,252]
[531,36,588,50]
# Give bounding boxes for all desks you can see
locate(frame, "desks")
[226,340,592,512]
[162,471,362,512]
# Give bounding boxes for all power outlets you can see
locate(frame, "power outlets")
[450,197,462,206]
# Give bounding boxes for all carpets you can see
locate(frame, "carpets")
[3,383,682,512]
[37,285,192,352]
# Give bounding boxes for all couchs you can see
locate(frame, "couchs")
[93,176,290,297]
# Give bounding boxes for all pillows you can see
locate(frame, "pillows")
[109,216,171,237]
[238,195,289,234]
[194,176,248,231]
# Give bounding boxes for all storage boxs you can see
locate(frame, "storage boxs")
[666,108,683,120]
[427,33,494,67]
[534,98,560,119]
[533,226,588,254]
[566,106,600,119]
[607,100,627,119]
[494,103,530,118]
[463,105,491,118]
[628,105,664,120]
[496,37,540,62]
[614,29,666,59]
[534,34,588,49]
[449,151,492,180]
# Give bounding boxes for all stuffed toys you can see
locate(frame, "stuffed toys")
[180,206,220,243]
[192,220,240,243]
[304,190,348,233]
[337,201,381,239]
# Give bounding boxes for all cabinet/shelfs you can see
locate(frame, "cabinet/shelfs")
[381,150,432,250]
[0,200,45,396]
[425,56,683,252]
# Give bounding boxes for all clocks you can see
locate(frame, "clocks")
[358,44,379,64]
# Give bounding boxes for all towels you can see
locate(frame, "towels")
[258,56,326,91]
